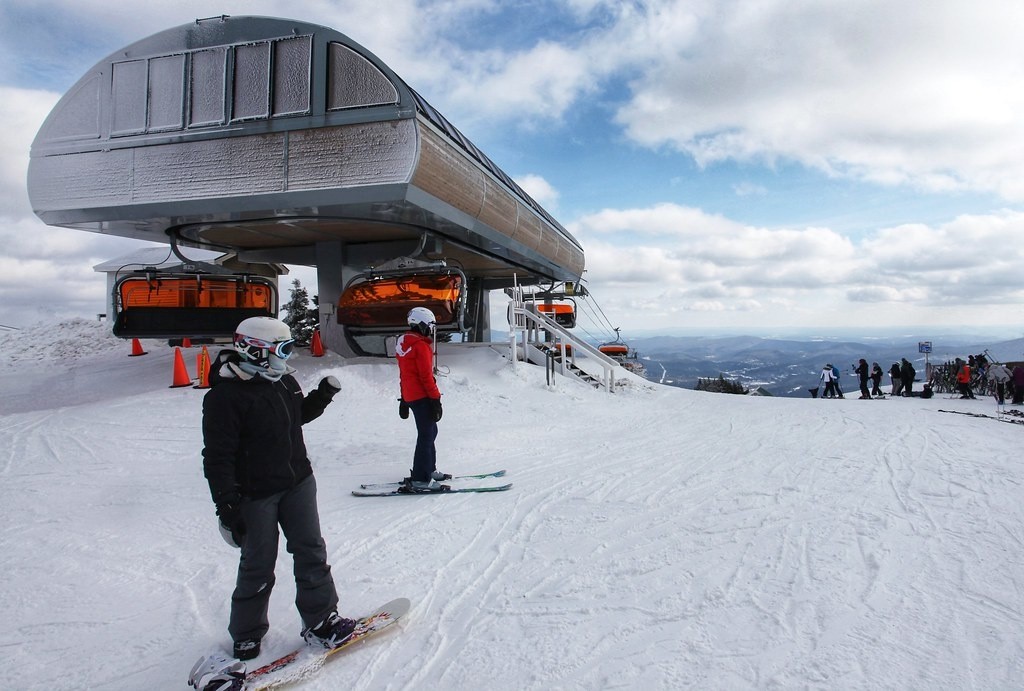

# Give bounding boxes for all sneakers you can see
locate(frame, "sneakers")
[300,611,356,649]
[412,478,450,491]
[233,637,261,661]
[410,469,452,481]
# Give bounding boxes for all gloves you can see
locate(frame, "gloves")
[431,398,442,422]
[216,496,243,547]
[318,376,341,407]
[397,398,409,419]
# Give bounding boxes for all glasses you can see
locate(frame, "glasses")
[275,339,294,359]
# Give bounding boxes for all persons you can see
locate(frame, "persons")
[820,364,843,398]
[854,358,916,399]
[202,317,357,662]
[952,354,1024,405]
[394,307,451,490]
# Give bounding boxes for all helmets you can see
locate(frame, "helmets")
[896,361,900,365]
[873,362,879,366]
[827,363,832,367]
[232,316,290,381]
[407,307,435,337]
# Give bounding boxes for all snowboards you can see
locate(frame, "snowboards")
[208,596,412,691]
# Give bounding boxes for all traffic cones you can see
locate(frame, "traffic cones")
[192,345,212,389]
[183,337,193,348]
[311,329,325,357]
[170,347,195,388]
[128,337,149,356]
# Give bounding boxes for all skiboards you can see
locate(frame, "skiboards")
[943,396,984,401]
[351,469,513,497]
[821,391,893,400]
